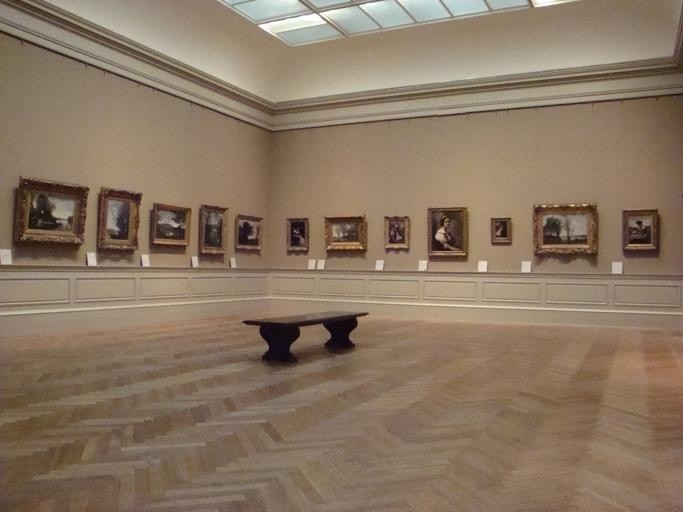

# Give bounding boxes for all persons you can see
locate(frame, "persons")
[496,223,507,239]
[434,214,464,252]
[636,219,648,239]
[390,225,404,243]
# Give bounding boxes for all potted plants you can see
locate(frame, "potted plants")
[234,213,263,251]
[151,201,191,247]
[97,186,142,251]
[12,175,89,250]
[198,203,229,254]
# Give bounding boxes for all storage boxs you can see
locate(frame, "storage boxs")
[242,309,372,361]
